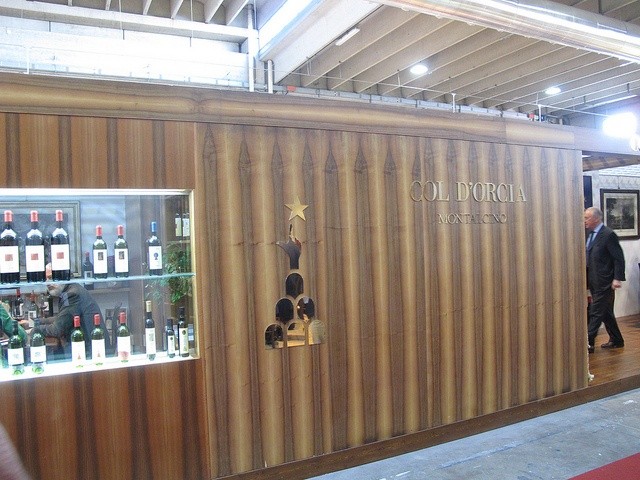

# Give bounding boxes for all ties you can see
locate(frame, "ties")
[589,231,594,246]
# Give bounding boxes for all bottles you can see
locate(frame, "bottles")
[90,313,106,366]
[114,225,129,277]
[3,287,53,327]
[177,307,190,357]
[143,301,156,361]
[0,211,21,284]
[30,318,46,374]
[50,210,71,282]
[116,312,131,363]
[24,210,46,283]
[145,222,163,276]
[83,253,94,290]
[8,320,24,375]
[92,225,108,279]
[166,318,175,358]
[70,315,86,368]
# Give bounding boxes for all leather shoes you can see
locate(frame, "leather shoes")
[588,344,594,353]
[600,340,623,348]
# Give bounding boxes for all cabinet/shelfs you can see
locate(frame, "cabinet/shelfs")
[0,187,198,382]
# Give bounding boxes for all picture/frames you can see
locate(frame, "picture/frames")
[600,188,640,241]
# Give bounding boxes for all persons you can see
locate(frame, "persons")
[583,206,626,352]
[18,261,111,358]
[0,300,27,371]
